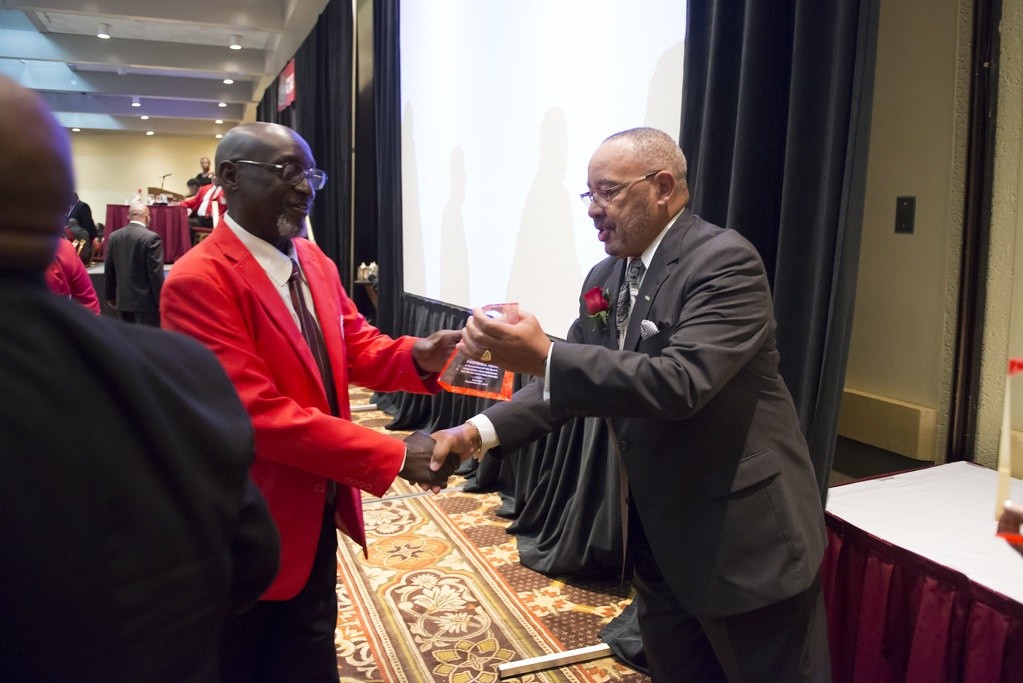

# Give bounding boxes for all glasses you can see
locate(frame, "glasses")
[233,159,329,191]
[580,171,659,208]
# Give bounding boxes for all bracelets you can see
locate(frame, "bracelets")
[543,358,547,372]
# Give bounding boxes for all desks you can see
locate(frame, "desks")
[824,460,1023,682]
[102,204,193,263]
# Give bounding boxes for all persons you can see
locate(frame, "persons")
[44,192,105,319]
[104,201,164,327]
[408,127,833,683]
[168,157,227,251]
[158,123,464,683]
[0,74,284,683]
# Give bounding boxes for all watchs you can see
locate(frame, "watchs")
[467,421,482,453]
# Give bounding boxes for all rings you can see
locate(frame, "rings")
[479,351,492,363]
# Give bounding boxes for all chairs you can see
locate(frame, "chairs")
[71,238,103,268]
[191,226,213,247]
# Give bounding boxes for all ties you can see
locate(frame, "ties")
[616,257,647,349]
[287,258,339,505]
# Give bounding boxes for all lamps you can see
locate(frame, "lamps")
[117,66,128,76]
[229,34,242,50]
[95,22,112,39]
[132,96,141,108]
[223,74,233,85]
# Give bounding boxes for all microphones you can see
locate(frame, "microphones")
[162,173,172,177]
